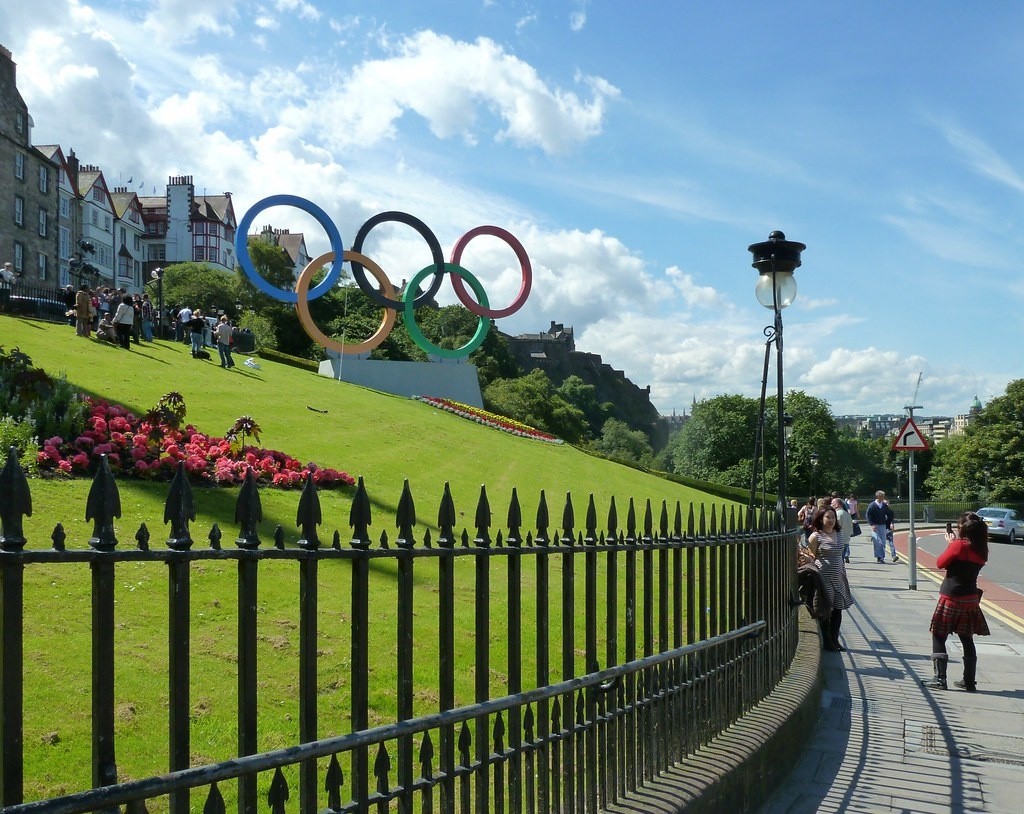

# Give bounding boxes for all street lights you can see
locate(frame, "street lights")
[745,230,807,521]
[810,451,820,498]
[151,267,165,339]
[895,462,902,498]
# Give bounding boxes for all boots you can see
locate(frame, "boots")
[953,656,978,692]
[830,615,847,652]
[819,614,836,652]
[920,652,948,690]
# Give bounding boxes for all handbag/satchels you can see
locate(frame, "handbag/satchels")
[113,320,119,327]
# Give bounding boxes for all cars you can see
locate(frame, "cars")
[975,507,1024,544]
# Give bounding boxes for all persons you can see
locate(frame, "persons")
[0,261,20,315]
[62,283,239,369]
[791,490,899,653]
[921,511,990,691]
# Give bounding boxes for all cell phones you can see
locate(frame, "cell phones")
[946,523,953,534]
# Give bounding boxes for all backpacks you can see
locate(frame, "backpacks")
[803,507,815,528]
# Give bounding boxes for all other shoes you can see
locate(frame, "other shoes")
[892,556,899,562]
[877,557,884,563]
[845,555,850,563]
